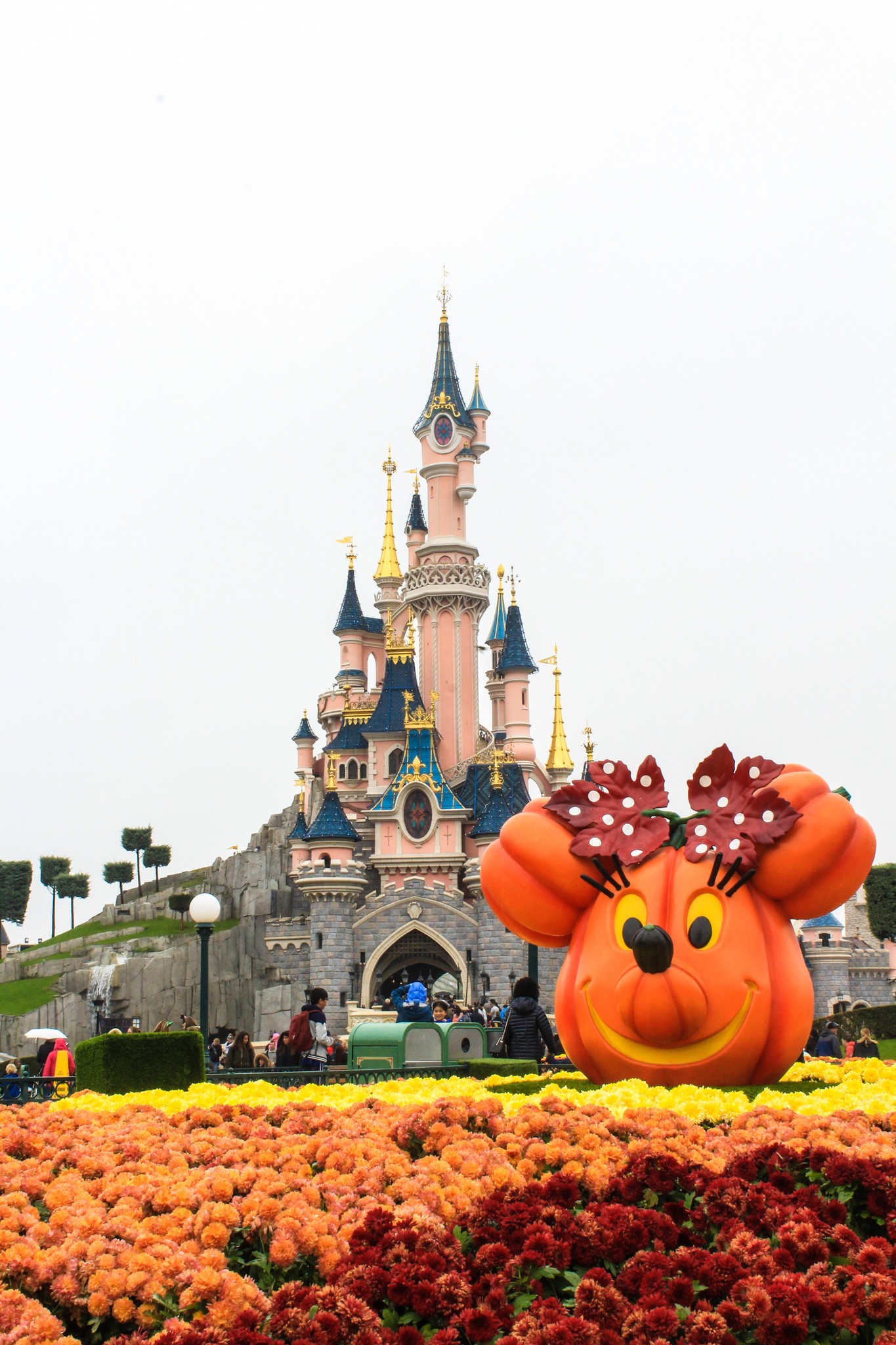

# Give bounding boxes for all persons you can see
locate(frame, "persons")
[370,994,406,1011]
[205,1030,347,1074]
[128,1026,140,1033]
[391,982,434,1023]
[0,1064,21,1107]
[805,1026,819,1057]
[43,1039,74,1099]
[180,1014,200,1031]
[153,1020,173,1033]
[489,977,555,1064]
[852,1028,880,1060]
[1,1059,22,1077]
[842,1034,856,1058]
[814,1021,842,1059]
[37,1038,54,1098]
[429,995,508,1028]
[108,1028,122,1034]
[541,1027,573,1065]
[290,987,335,1086]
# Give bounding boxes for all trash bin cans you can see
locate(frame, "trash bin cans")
[346,1020,491,1069]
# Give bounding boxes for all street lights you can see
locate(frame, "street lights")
[188,892,220,1064]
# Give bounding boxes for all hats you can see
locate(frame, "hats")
[449,1004,456,1008]
[228,1033,234,1039]
[385,998,390,1004]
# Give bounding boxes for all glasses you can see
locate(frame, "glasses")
[227,1036,231,1038]
[324,998,329,1001]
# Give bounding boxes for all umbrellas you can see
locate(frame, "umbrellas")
[434,991,452,995]
[0,1052,17,1065]
[24,1028,67,1040]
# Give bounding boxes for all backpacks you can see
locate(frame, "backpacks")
[458,1016,465,1022]
[289,1010,322,1052]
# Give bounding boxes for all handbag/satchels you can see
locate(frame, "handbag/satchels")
[490,1036,505,1058]
[220,1057,226,1066]
